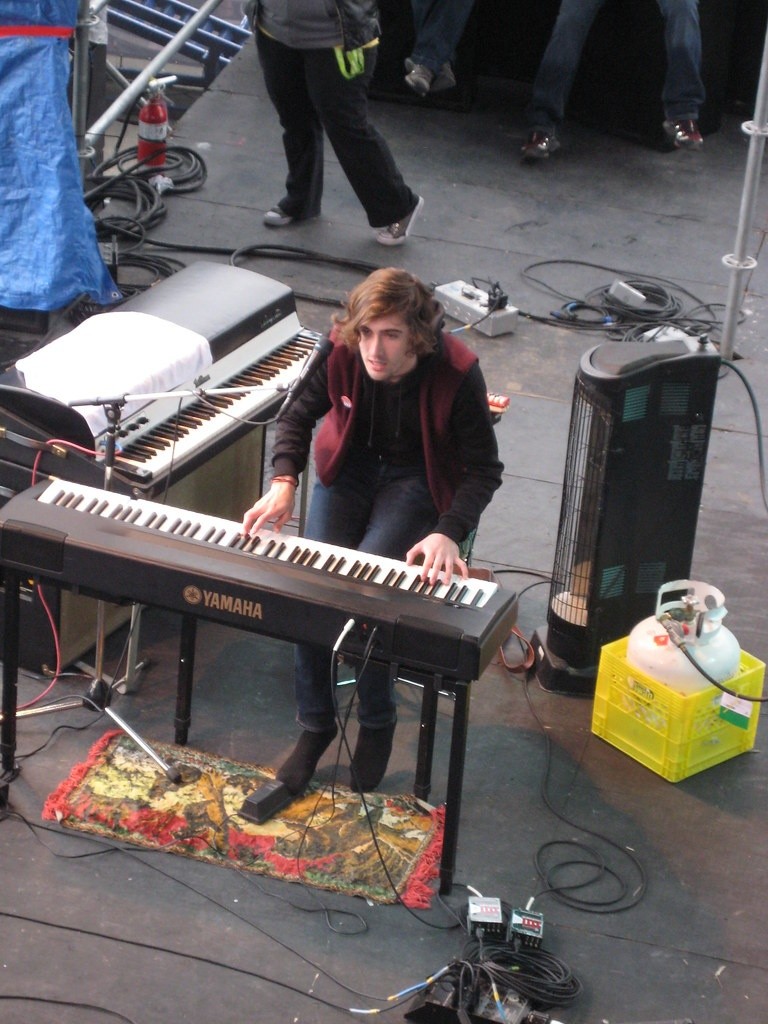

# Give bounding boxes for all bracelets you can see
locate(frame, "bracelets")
[271,477,299,490]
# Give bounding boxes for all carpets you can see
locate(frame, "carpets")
[55,726,442,908]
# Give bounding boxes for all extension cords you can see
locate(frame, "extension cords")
[433,280,519,336]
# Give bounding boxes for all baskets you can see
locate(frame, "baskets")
[590,635,766,784]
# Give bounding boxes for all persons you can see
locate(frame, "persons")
[521,0,704,160]
[405,0,473,97]
[243,267,505,801]
[240,1,424,246]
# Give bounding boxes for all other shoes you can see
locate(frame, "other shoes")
[431,63,458,94]
[404,56,433,98]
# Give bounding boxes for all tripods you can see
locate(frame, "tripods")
[0,383,289,781]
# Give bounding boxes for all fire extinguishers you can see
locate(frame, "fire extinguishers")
[137,76,178,178]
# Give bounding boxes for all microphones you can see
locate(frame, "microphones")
[277,339,334,419]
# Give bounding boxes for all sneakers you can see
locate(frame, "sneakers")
[263,203,293,227]
[377,195,424,246]
[518,131,560,161]
[662,117,704,151]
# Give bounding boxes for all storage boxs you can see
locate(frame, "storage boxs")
[590,636,765,786]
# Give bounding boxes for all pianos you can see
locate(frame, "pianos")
[0,260,326,678]
[0,471,521,684]
